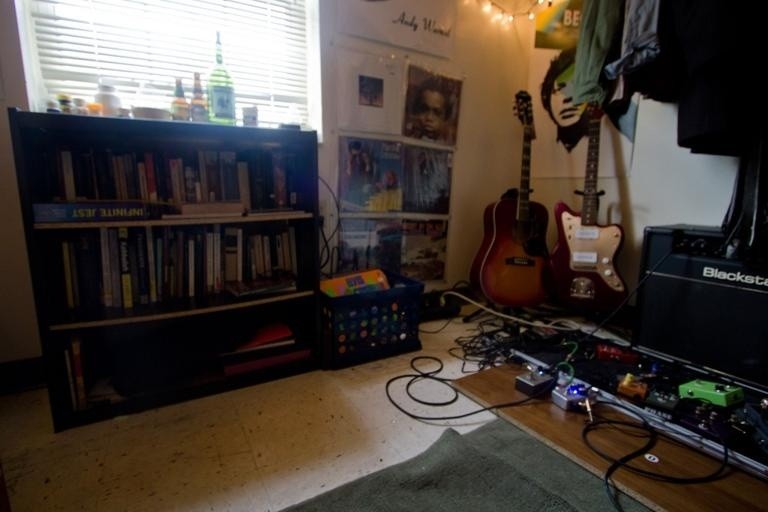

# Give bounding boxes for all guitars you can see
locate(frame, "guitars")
[469,90,549,309]
[543,100,630,324]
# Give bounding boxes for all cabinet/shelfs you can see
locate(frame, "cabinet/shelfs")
[7,107,320,437]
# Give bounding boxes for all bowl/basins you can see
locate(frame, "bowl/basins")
[131,106,172,120]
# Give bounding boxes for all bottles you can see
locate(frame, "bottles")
[209,30,238,126]
[46,83,120,117]
[172,71,209,121]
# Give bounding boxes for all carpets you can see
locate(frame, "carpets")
[271,418,660,511]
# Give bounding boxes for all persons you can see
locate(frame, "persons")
[539,49,620,152]
[414,77,453,146]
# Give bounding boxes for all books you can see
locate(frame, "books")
[70,340,88,407]
[62,348,76,408]
